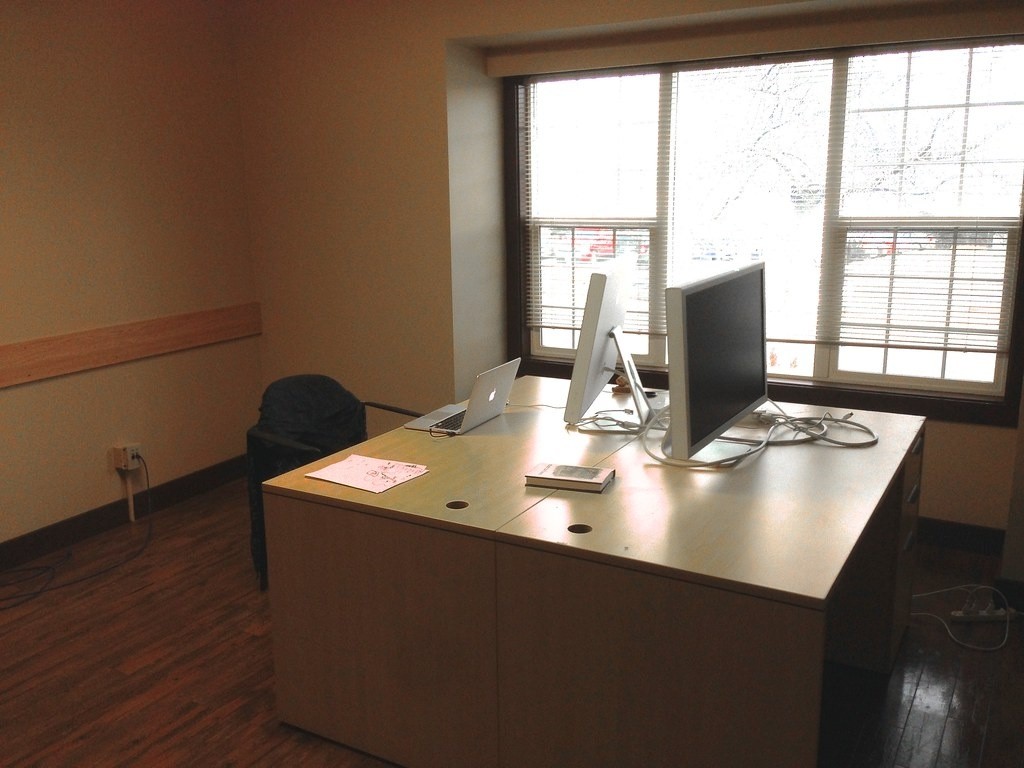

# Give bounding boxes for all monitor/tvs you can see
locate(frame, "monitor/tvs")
[657,259,767,467]
[565,272,649,433]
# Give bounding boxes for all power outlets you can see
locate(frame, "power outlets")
[113,443,142,471]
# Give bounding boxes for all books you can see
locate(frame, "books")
[525,463,615,493]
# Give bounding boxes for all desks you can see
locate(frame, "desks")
[263,375,926,768]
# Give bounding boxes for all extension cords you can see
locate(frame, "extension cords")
[950,608,1017,622]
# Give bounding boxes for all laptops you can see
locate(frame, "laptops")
[403,357,521,434]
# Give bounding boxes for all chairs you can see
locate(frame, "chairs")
[247,375,366,588]
